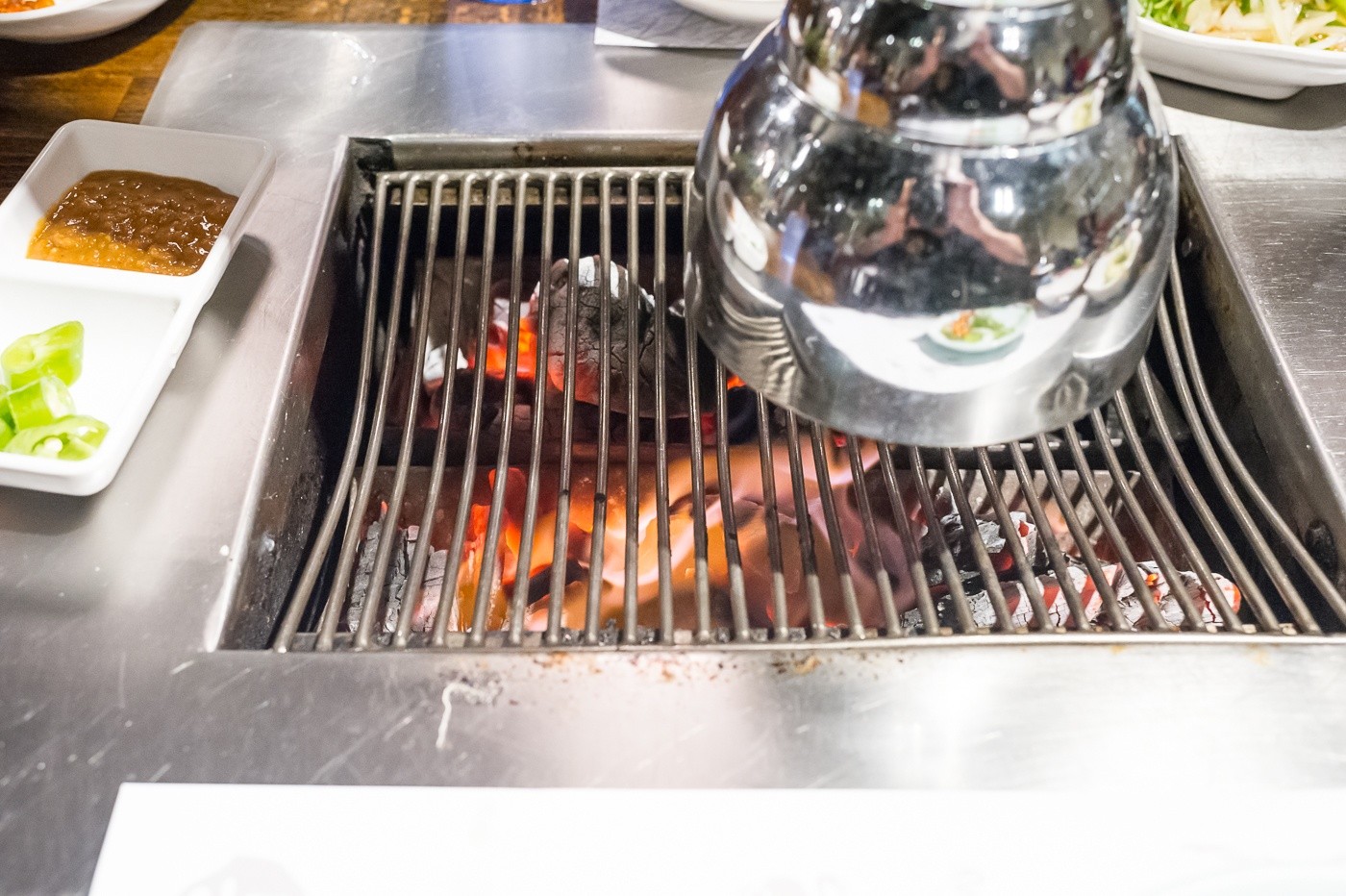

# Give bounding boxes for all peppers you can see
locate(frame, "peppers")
[0,319,109,467]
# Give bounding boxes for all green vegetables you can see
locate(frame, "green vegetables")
[1134,0,1346,56]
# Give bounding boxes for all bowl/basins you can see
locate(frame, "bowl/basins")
[1129,2,1345,100]
[1035,231,1143,308]
[0,0,167,42]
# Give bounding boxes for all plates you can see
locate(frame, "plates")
[715,181,768,271]
[925,308,1026,353]
[1,116,278,496]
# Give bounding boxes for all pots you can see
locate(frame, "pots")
[682,0,1180,450]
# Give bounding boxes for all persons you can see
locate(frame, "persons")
[695,2,1160,298]
